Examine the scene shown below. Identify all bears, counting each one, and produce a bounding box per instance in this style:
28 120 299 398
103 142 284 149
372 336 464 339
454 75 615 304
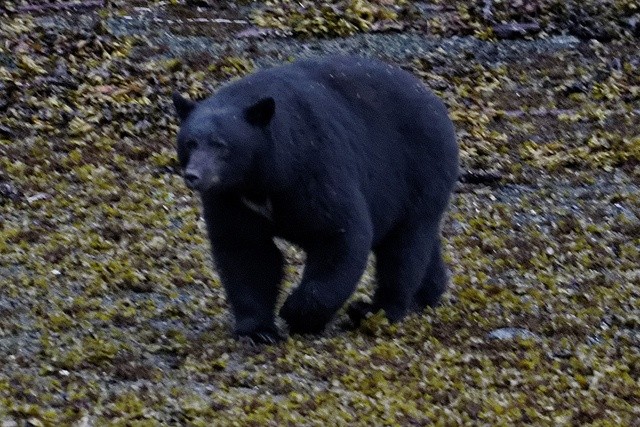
169 51 461 352
